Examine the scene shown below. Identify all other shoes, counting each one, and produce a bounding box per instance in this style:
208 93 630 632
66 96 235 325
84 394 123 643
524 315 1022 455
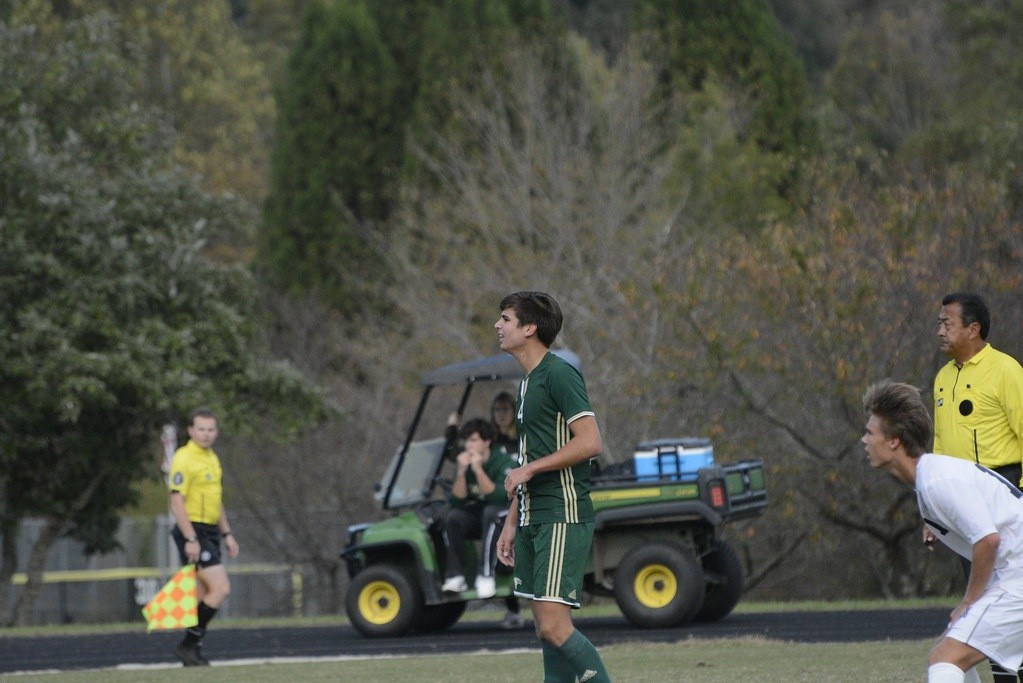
171 641 210 667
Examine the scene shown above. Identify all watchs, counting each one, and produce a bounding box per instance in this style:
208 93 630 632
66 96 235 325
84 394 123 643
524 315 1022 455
185 535 197 542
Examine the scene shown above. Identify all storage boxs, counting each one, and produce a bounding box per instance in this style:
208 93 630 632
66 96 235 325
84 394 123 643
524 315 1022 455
633 438 716 482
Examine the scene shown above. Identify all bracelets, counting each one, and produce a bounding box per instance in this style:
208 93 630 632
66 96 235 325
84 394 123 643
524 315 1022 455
222 531 232 539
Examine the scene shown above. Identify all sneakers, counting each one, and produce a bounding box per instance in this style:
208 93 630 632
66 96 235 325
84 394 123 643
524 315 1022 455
475 574 496 600
497 613 520 628
441 574 468 593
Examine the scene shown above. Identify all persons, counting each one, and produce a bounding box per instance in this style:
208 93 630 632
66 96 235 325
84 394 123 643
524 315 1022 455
495 291 612 683
858 378 1023 683
912 288 1023 683
435 391 528 630
167 406 238 669
